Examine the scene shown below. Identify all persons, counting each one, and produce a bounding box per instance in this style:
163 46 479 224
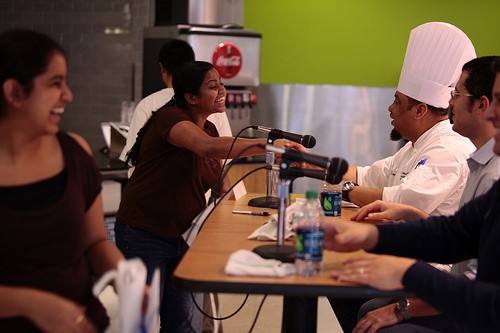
119 35 235 247
275 55 500 333
113 60 304 332
0 26 153 333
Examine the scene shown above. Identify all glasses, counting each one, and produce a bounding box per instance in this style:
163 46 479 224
449 90 473 99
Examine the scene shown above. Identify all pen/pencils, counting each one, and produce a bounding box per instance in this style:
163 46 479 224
232 210 271 216
414 159 425 170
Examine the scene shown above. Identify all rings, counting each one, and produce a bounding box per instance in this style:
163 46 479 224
74 313 84 324
359 267 364 275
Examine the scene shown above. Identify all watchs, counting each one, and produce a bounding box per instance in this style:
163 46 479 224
396 297 410 317
342 181 360 203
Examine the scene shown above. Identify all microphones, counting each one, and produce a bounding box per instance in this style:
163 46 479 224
265 143 348 173
266 163 343 185
252 124 316 148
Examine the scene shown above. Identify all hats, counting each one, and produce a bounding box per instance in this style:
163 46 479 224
396 22 478 110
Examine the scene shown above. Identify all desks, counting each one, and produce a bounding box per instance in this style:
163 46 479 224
175 162 417 333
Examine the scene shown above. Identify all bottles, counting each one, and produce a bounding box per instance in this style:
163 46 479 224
294 190 325 278
320 180 343 219
